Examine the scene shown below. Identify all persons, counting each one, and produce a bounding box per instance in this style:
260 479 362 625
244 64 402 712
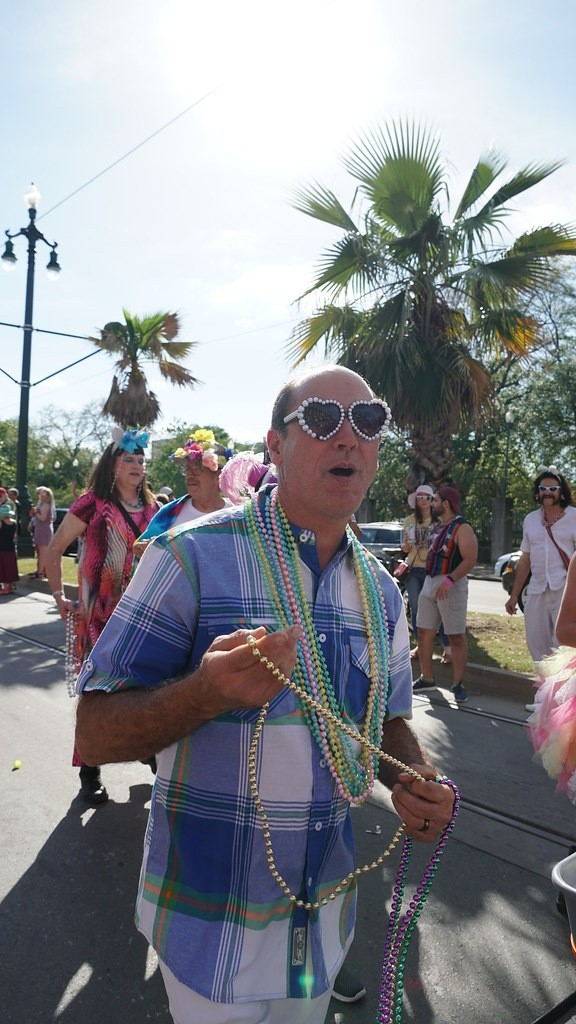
0 487 21 594
331 965 366 1002
28 486 56 579
524 552 576 807
505 471 575 714
73 362 456 1024
399 483 452 663
412 487 477 703
43 428 157 803
130 429 235 560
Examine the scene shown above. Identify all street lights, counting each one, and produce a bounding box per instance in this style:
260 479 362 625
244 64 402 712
0 179 62 559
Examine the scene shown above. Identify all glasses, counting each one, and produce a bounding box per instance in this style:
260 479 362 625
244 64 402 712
416 495 432 501
178 462 210 476
539 486 561 492
282 397 393 443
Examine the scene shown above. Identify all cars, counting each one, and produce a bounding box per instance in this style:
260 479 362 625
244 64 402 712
495 550 532 614
14 508 78 556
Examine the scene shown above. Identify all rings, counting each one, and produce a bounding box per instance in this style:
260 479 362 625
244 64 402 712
419 820 429 832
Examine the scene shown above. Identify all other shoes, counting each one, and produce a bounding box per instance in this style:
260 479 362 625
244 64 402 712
526 703 538 712
78 762 110 803
27 571 45 579
410 648 418 658
0 587 14 595
331 963 366 1003
441 652 452 663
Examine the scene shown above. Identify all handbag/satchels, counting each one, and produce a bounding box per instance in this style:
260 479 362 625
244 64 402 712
393 563 412 581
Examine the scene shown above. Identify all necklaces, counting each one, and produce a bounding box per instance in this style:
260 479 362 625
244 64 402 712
540 507 563 527
65 610 78 698
243 484 392 803
378 776 461 1023
430 517 452 553
244 634 428 912
414 518 432 562
121 494 143 509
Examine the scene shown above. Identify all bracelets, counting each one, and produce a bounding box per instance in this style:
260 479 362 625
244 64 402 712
52 590 64 597
443 577 453 587
447 575 455 582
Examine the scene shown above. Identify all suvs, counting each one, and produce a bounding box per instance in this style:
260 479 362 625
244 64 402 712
356 521 403 570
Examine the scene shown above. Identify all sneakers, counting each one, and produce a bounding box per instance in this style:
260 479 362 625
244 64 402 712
451 680 467 702
412 675 437 691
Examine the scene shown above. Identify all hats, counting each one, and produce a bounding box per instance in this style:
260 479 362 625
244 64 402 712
216 449 279 506
0 504 15 515
166 428 233 468
159 486 174 495
407 484 437 508
0 487 7 494
438 486 461 513
